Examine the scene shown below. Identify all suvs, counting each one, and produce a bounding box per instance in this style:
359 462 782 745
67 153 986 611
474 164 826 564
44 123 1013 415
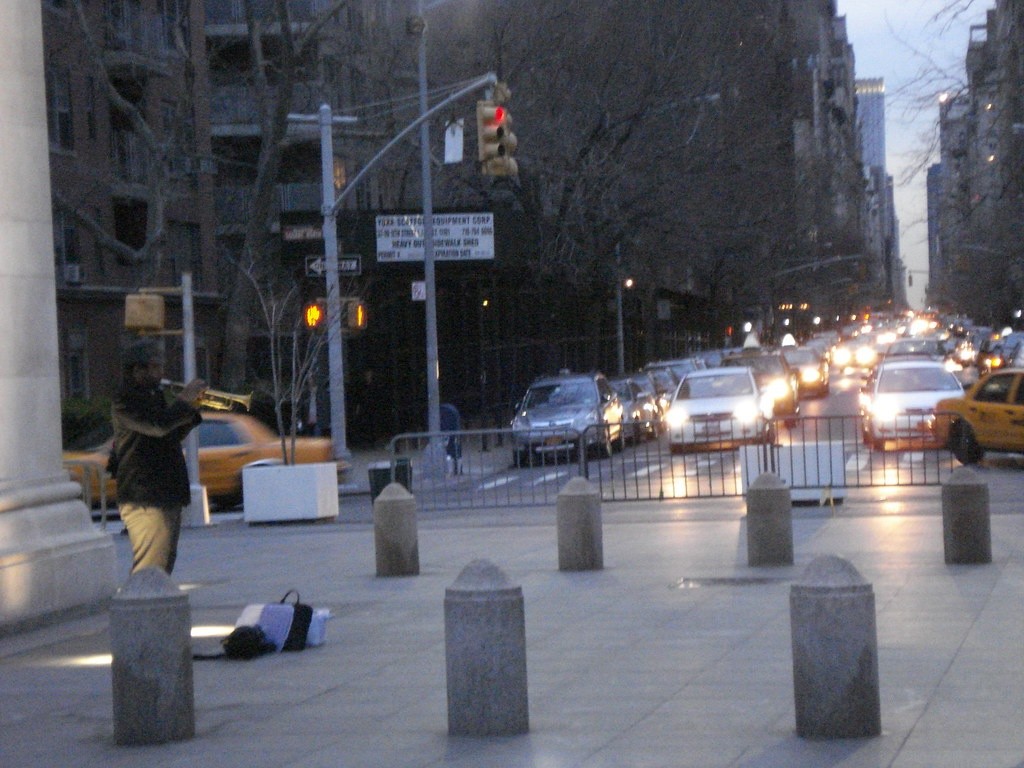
511 369 625 468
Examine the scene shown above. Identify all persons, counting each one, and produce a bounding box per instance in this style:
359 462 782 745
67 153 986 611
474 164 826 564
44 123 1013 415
441 398 467 475
105 338 214 580
480 364 504 453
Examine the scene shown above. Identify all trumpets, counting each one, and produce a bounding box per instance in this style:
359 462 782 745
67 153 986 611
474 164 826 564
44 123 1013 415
158 375 255 414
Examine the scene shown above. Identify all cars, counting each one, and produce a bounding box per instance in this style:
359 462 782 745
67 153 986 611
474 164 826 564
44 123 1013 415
64 408 333 510
928 366 1024 465
664 366 774 451
609 310 1024 441
856 361 965 449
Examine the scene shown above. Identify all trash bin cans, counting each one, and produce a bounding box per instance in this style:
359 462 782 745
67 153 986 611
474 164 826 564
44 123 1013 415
368 457 413 508
424 404 463 476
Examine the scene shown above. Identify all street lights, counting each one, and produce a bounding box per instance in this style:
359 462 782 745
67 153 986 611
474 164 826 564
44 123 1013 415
611 89 724 374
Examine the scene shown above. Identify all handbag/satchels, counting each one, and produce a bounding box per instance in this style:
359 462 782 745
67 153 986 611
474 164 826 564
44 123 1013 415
258 590 312 649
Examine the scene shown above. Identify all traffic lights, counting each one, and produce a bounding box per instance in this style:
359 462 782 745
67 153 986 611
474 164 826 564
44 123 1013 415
304 303 323 331
474 99 506 165
347 302 367 329
481 112 520 178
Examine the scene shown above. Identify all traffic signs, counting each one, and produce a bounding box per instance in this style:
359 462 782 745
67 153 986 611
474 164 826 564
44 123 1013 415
303 252 363 277
